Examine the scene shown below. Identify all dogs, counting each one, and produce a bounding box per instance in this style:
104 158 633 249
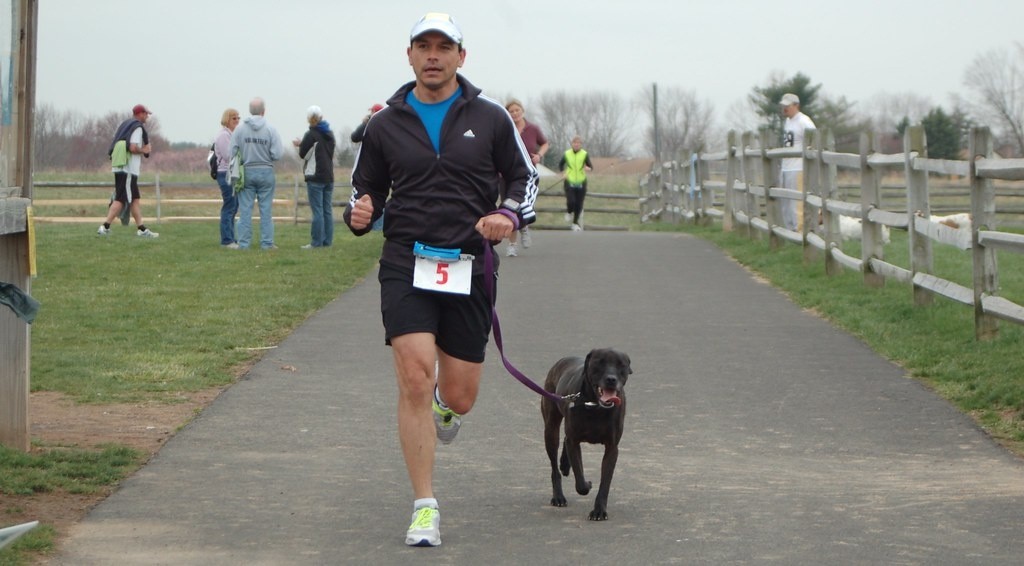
541 348 633 521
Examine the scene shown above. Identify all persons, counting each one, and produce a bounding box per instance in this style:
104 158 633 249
777 93 817 234
558 134 593 231
230 97 282 249
214 108 238 248
342 13 538 547
499 98 549 257
292 106 336 248
97 104 159 238
350 104 383 231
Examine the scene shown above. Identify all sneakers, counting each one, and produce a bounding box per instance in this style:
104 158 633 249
520 230 531 248
505 246 517 257
97 225 113 236
138 228 160 238
405 507 442 546
431 380 462 445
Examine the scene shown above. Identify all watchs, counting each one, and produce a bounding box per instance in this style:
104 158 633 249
499 198 521 214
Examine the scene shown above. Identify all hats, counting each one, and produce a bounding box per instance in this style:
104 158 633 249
134 104 153 115
779 93 799 105
409 13 463 44
369 104 383 111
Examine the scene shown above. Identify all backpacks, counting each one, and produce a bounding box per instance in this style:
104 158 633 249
208 143 222 180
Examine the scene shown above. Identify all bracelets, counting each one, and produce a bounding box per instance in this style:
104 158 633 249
534 152 541 157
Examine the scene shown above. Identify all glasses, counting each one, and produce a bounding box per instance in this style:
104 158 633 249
232 117 240 120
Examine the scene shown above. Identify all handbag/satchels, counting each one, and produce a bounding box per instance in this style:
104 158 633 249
111 141 130 169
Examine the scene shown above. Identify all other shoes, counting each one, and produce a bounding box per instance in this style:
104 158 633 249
564 213 572 223
572 223 580 231
222 243 239 249
300 245 312 249
